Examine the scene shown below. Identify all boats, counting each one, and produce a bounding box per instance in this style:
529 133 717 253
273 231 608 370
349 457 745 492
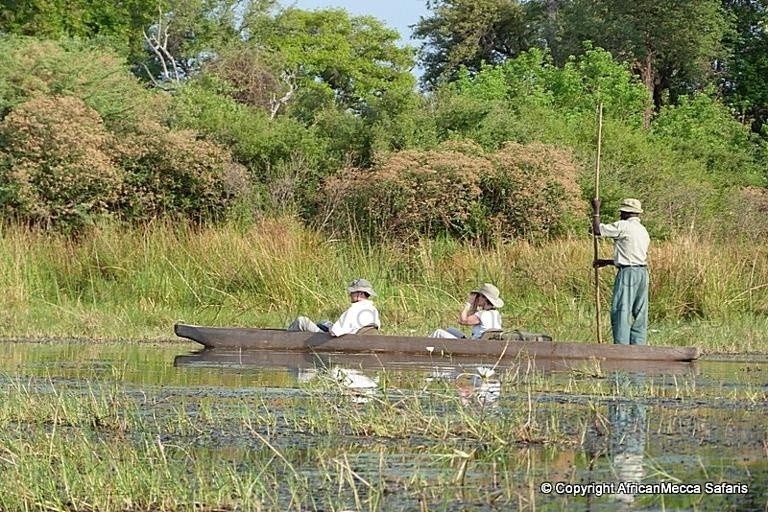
173 320 704 367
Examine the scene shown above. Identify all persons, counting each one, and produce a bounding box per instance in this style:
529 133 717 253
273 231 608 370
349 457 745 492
288 279 380 338
591 198 650 346
429 283 504 339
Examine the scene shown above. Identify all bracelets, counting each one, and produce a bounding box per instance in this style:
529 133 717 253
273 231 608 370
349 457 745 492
593 213 599 217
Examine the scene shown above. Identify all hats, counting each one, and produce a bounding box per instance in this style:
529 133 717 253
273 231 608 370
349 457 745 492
618 199 644 214
470 283 504 308
347 279 377 296
